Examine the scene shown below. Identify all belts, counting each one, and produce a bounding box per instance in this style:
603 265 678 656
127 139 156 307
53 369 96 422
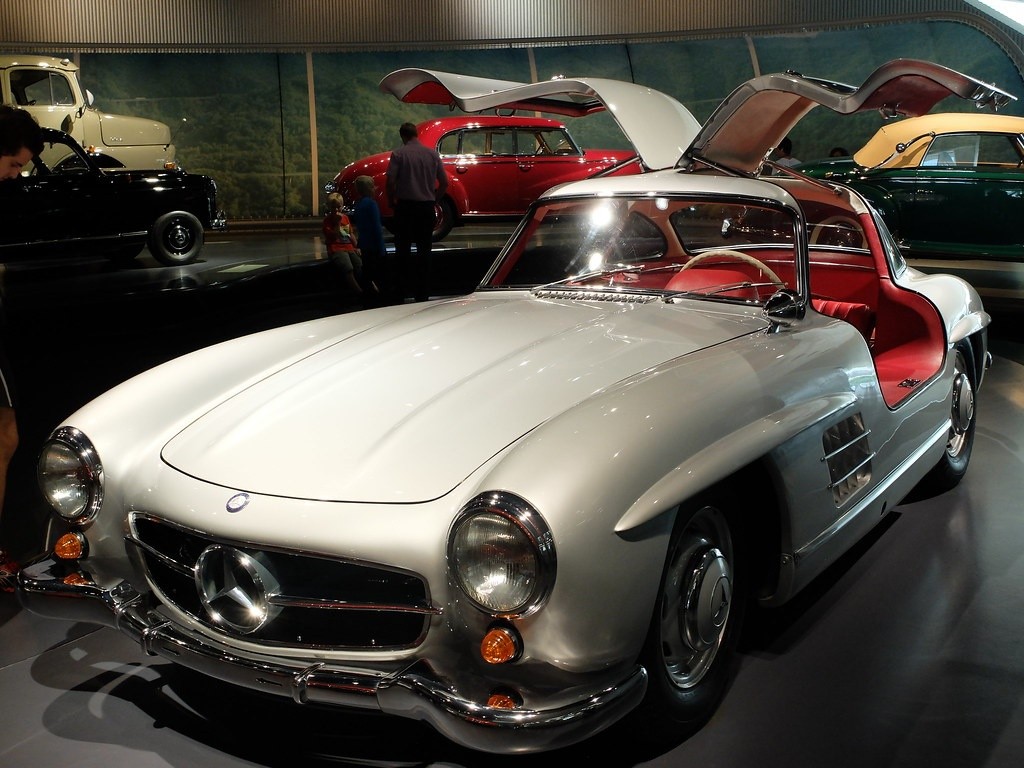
398 199 436 205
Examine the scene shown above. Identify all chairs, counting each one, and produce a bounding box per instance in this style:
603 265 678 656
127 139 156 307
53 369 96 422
811 299 876 345
937 152 955 166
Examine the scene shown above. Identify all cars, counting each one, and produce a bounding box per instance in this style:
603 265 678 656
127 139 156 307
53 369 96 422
0 53 177 174
323 112 1024 306
0 126 228 278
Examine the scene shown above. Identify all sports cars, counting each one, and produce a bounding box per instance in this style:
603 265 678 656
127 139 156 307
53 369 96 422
25 57 1020 763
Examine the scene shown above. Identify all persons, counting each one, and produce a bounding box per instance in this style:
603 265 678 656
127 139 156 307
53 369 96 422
829 147 850 157
345 175 386 296
772 138 802 175
0 104 68 592
385 123 448 303
323 192 363 294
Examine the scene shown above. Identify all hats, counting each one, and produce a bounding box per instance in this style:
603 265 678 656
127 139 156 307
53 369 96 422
355 175 373 193
328 192 343 206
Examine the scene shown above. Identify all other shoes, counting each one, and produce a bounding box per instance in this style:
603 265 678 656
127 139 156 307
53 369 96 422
0 549 18 592
